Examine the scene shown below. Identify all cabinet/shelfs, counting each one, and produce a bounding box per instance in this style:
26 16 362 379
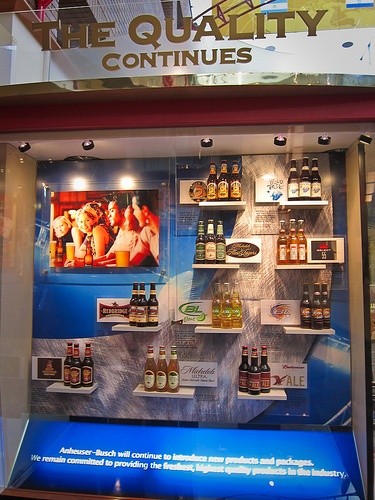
46 200 336 401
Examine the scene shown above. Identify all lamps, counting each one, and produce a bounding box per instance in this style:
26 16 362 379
201 137 213 147
274 135 287 145
18 142 30 153
358 132 373 145
82 140 94 150
318 132 334 146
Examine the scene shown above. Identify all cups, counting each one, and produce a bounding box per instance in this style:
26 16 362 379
66 242 75 261
115 245 130 267
50 241 57 259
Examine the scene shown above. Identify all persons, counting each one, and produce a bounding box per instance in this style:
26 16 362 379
52 192 159 266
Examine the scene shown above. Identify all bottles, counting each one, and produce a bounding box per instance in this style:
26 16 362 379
57 239 64 263
144 345 180 392
277 218 307 265
64 342 94 387
207 160 242 201
300 282 330 330
85 236 93 266
287 157 322 201
128 282 159 326
195 218 226 264
238 346 270 395
213 282 243 329
